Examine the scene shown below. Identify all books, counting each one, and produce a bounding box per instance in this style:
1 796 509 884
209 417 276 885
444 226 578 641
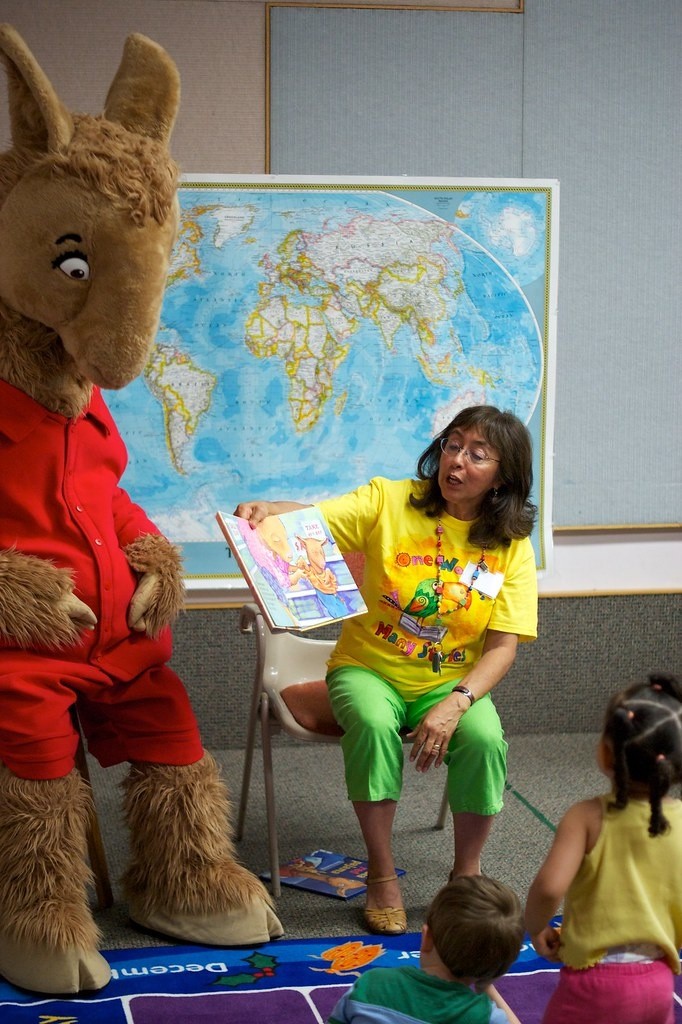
215 504 368 634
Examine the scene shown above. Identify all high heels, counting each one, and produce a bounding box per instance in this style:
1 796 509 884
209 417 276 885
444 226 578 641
362 874 408 935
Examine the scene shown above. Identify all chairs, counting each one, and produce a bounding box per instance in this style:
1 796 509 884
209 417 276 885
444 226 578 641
234 602 448 898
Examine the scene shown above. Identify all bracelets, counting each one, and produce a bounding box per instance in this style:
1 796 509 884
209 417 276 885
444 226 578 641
452 686 476 707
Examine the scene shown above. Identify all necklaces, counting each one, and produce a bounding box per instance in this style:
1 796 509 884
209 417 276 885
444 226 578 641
432 507 487 644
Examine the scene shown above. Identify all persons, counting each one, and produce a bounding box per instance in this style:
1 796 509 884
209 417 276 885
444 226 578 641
523 668 682 1024
318 875 526 1024
236 402 540 938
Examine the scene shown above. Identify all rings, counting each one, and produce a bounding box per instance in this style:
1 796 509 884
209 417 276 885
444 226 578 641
433 745 441 751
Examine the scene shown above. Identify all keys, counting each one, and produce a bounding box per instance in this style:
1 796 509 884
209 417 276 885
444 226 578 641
437 652 443 677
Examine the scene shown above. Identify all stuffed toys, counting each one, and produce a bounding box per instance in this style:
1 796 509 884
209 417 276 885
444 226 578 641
0 23 288 1002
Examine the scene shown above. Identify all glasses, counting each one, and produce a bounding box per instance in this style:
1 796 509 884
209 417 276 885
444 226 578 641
440 437 501 465
